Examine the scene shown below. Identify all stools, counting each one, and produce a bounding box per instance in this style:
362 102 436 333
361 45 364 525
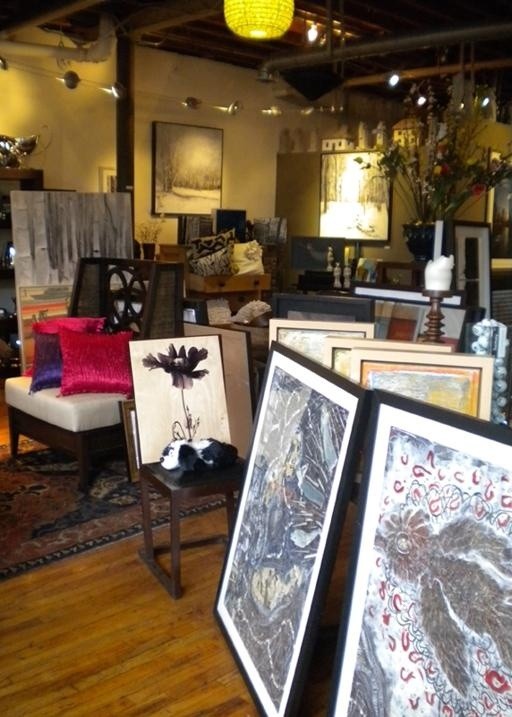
138 453 248 598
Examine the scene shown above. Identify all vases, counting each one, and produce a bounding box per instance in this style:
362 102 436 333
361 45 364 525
403 220 436 260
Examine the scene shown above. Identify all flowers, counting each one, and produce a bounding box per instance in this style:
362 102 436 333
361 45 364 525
355 117 512 222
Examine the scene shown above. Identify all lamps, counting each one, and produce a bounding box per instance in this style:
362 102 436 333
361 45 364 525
390 75 400 86
307 24 319 41
416 97 426 106
224 0 295 38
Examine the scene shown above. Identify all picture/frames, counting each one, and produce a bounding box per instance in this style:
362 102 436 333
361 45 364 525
322 390 512 716
10 191 134 375
324 336 453 380
319 149 394 241
130 333 234 468
152 121 223 215
213 342 369 717
351 348 494 422
269 318 374 368
375 299 474 354
272 293 375 322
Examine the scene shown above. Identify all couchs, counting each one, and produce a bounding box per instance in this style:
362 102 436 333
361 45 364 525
5 256 184 494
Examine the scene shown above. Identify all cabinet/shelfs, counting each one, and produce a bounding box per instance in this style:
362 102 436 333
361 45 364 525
0 169 44 375
